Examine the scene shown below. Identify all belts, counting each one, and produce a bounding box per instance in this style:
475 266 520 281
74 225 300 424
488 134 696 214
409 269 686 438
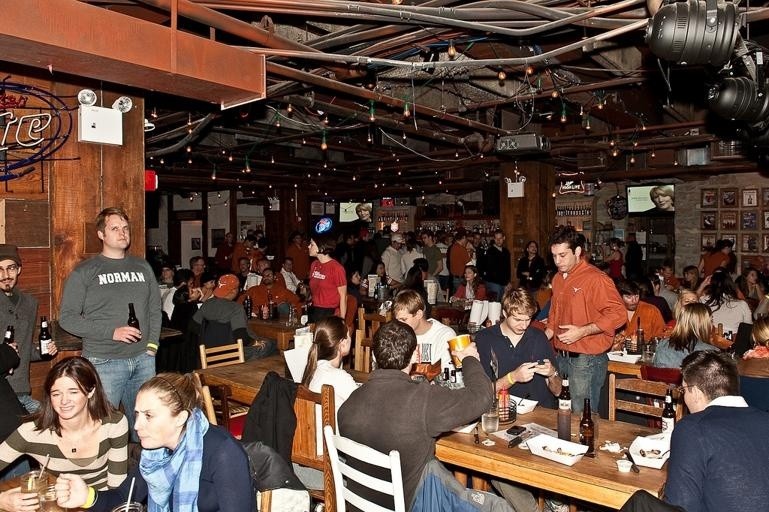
554 347 584 360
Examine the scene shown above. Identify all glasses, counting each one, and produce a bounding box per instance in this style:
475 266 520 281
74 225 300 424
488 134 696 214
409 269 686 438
675 383 696 394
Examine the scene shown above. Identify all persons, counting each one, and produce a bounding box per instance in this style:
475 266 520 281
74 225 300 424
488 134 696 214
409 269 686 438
472 285 572 512
335 320 516 512
53 371 258 511
639 183 674 212
1 355 129 491
58 205 163 444
1 242 59 419
148 215 768 419
0 485 42 512
0 337 33 482
290 314 365 492
347 203 373 222
618 348 768 511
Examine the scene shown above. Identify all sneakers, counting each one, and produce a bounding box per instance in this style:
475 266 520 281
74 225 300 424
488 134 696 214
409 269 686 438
543 498 570 512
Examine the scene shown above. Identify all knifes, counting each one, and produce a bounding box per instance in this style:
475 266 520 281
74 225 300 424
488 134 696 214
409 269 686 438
474 426 479 444
508 430 532 448
624 451 640 473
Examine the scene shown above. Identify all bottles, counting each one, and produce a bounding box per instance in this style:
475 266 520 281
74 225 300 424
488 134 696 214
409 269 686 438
4 325 18 376
584 238 591 251
579 398 595 452
128 303 142 343
557 402 571 442
372 281 392 301
556 203 591 217
717 323 733 352
38 315 53 360
661 389 675 434
288 304 309 326
443 368 462 385
592 254 596 261
378 211 408 221
420 221 499 234
498 385 511 421
244 294 252 319
558 374 571 412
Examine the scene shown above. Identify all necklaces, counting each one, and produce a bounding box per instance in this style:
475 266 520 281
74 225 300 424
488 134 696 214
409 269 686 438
51 418 103 452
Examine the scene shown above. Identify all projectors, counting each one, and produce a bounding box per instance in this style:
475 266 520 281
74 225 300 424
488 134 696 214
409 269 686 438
497 134 552 155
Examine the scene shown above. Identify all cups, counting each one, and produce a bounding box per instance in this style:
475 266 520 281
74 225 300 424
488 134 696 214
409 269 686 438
466 299 502 333
616 459 633 473
19 471 51 512
625 328 661 365
110 502 147 512
447 335 470 368
38 484 67 512
481 412 499 435
259 302 278 320
580 411 599 448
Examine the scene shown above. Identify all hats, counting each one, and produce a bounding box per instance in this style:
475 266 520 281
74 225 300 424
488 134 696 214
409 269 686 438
0 242 23 268
161 263 177 276
390 232 406 244
212 273 241 299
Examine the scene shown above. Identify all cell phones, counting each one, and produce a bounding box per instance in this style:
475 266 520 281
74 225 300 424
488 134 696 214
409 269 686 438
507 424 527 435
531 360 544 370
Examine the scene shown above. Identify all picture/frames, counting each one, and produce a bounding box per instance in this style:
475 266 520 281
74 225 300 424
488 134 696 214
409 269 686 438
695 184 769 276
